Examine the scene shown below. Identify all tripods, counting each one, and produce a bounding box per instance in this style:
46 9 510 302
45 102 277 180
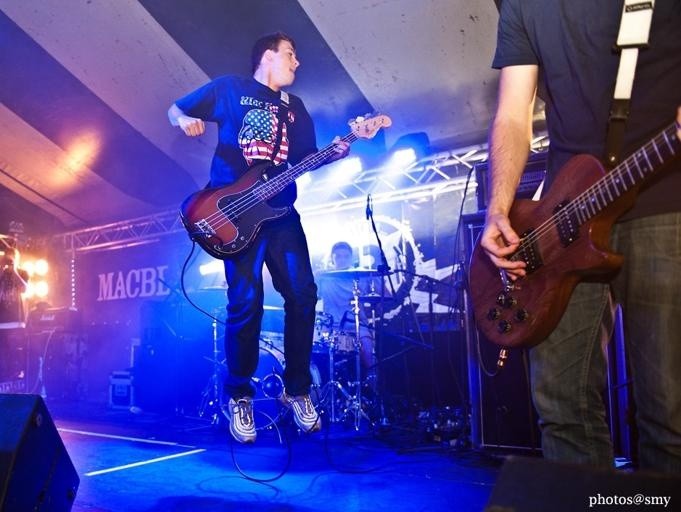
199 296 375 431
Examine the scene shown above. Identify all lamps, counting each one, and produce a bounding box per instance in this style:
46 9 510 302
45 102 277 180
330 144 372 176
389 132 432 166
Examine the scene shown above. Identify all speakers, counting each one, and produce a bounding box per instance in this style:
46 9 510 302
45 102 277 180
374 310 471 406
0 393 81 511
476 330 537 449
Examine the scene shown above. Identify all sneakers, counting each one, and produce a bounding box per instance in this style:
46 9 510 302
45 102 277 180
229 396 257 444
280 387 321 433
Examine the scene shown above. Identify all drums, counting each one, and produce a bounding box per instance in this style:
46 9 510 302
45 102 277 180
259 305 285 339
322 330 356 356
211 338 323 433
310 308 333 355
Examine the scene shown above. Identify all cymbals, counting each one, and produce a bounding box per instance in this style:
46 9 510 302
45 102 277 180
351 293 393 304
321 269 379 277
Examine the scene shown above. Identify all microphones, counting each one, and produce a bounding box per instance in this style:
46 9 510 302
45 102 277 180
365 197 371 220
496 346 511 371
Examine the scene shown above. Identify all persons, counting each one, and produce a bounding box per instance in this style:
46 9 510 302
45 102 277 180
316 242 390 380
167 34 350 443
0 247 29 378
481 0 681 475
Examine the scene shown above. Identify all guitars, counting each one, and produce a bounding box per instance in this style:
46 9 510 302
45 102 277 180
467 116 681 349
180 112 393 260
